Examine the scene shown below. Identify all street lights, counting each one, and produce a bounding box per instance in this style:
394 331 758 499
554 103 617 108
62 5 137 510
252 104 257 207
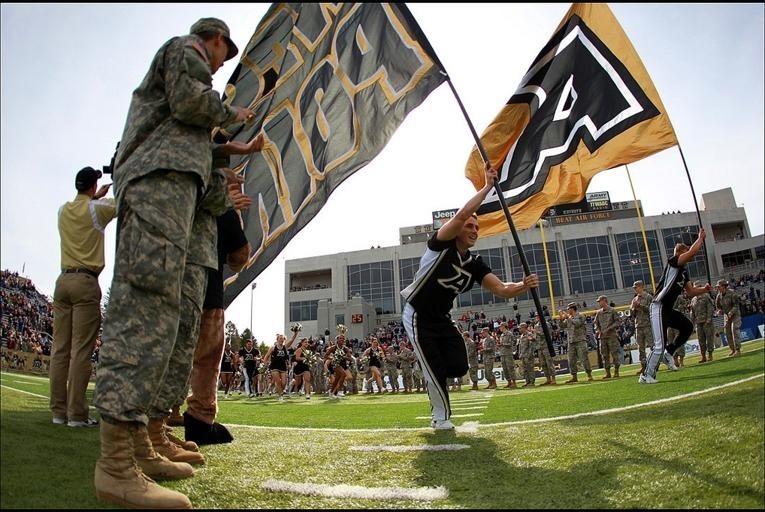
248 281 258 349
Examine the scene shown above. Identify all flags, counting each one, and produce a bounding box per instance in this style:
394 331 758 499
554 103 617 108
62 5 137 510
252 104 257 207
211 2 449 310
464 1 678 240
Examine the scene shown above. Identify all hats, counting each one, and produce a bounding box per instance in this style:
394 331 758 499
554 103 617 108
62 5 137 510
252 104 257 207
479 278 731 334
188 17 239 61
74 166 102 191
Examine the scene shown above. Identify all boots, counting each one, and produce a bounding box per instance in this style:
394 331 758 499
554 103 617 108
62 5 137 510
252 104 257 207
147 419 206 466
94 416 194 509
159 420 200 453
469 347 743 391
128 420 193 481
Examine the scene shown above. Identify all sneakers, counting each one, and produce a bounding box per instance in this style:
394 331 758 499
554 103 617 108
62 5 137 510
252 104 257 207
662 352 678 371
638 372 659 385
52 414 100 427
430 417 456 431
